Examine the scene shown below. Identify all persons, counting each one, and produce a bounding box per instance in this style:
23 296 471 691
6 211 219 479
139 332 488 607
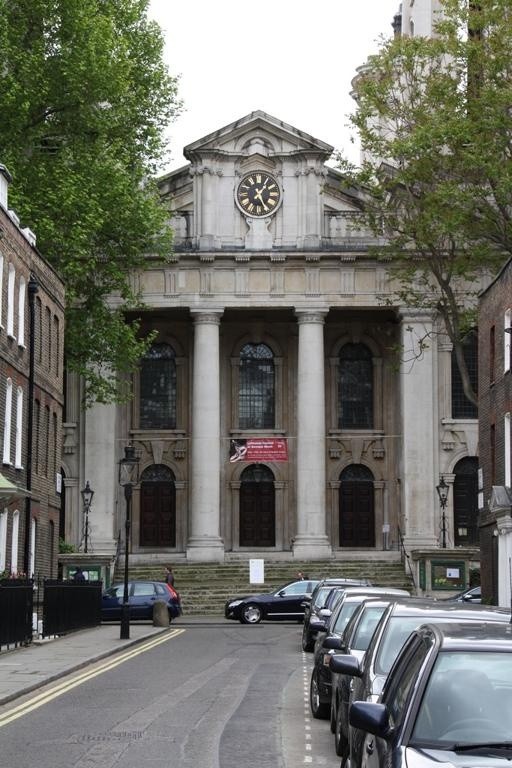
295 571 305 580
75 568 85 579
164 566 175 587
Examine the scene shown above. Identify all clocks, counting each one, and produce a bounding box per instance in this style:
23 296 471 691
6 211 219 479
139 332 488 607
232 168 284 220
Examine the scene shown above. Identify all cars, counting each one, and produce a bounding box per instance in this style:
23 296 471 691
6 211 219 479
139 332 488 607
101 580 183 624
320 600 395 758
222 576 373 625
445 586 482 603
346 623 512 767
301 582 412 719
330 599 511 768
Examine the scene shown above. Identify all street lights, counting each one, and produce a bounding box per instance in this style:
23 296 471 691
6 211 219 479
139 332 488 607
80 480 94 553
117 441 141 639
435 475 450 548
